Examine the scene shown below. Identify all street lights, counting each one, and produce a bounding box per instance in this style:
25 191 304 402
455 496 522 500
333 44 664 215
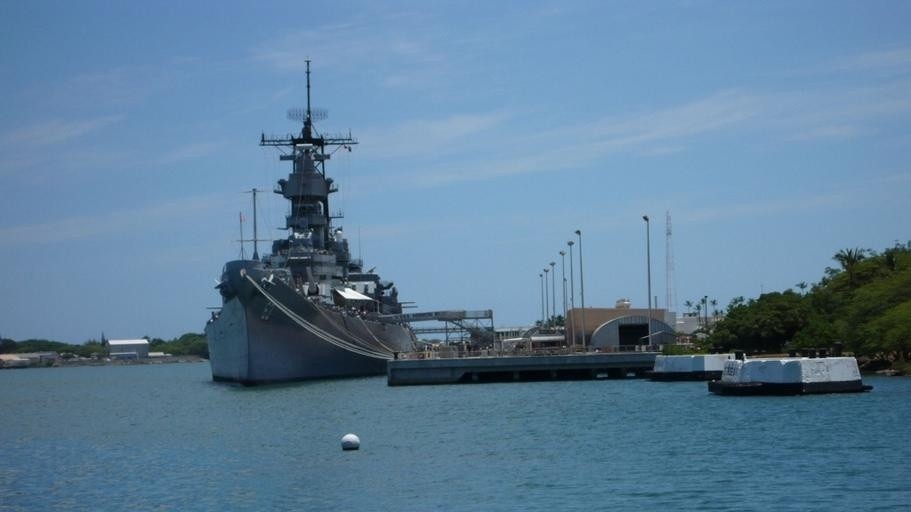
642 215 653 350
538 229 587 351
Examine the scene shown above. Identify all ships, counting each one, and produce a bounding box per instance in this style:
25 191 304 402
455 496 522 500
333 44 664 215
203 57 425 385
